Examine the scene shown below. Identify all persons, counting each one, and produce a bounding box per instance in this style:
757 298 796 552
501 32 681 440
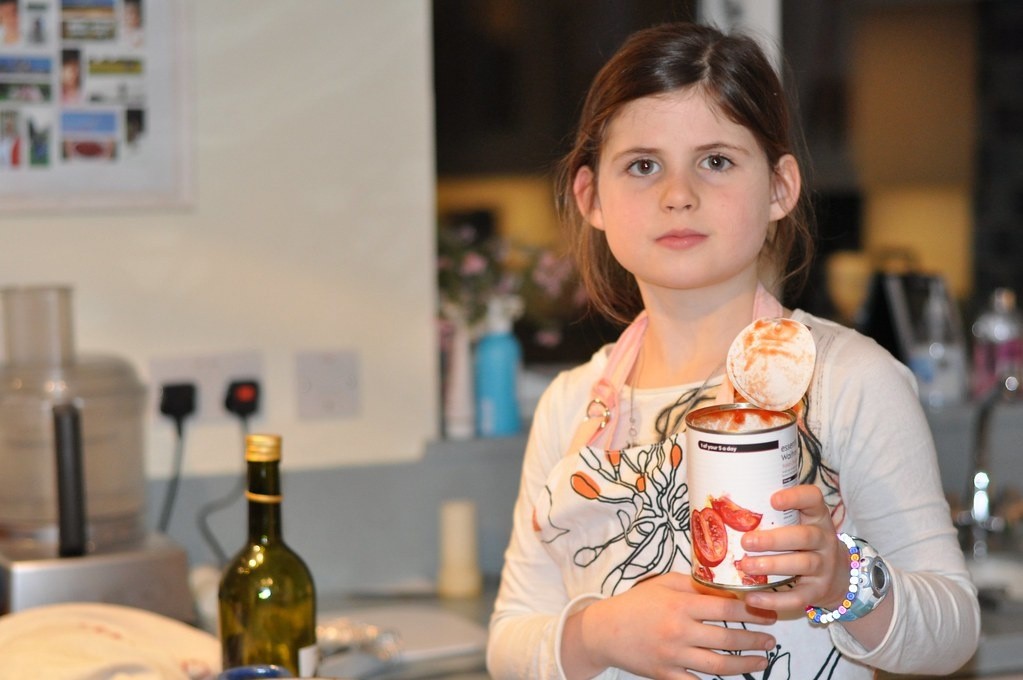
485 24 981 680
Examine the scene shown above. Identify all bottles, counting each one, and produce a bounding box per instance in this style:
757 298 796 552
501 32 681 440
909 277 965 403
218 435 316 680
474 294 520 434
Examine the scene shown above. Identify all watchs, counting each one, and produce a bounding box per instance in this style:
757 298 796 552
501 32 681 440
821 535 891 621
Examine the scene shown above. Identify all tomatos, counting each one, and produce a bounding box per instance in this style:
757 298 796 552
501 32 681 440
690 495 764 568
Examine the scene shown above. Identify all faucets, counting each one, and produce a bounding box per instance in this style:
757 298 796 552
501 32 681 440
962 370 1020 560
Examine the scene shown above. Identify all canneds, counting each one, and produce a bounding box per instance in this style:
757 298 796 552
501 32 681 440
684 400 801 595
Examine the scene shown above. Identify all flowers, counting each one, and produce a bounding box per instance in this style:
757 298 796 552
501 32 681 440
437 228 592 352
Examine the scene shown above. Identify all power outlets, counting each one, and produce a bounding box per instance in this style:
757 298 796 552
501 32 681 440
154 349 263 430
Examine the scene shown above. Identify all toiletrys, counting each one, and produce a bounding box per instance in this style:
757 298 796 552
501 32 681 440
473 292 526 437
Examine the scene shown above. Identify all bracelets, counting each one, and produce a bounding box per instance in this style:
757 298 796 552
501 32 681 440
804 533 860 624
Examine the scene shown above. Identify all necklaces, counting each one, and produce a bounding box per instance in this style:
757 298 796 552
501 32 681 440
630 341 726 447
